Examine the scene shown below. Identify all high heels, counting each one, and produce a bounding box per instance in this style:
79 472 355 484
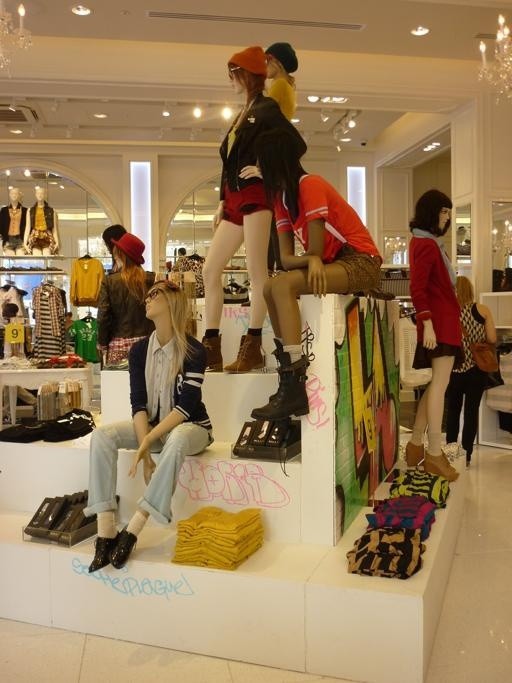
406 442 426 466
424 451 459 481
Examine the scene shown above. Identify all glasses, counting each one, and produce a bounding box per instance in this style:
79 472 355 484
145 288 159 299
228 66 240 79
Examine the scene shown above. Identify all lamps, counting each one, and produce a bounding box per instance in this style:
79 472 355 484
491 215 512 270
319 111 357 134
0 0 34 80
477 13 512 108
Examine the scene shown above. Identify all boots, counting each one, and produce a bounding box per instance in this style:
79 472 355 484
251 339 310 419
201 333 222 372
225 334 265 373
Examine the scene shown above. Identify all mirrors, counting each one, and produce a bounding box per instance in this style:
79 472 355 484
381 230 410 265
452 192 472 283
489 199 512 292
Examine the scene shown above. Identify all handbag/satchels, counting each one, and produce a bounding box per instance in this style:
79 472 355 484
470 342 499 373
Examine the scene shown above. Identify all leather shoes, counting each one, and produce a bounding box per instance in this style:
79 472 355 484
109 524 137 569
88 531 119 573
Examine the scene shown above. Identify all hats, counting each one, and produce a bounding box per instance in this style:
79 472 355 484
268 42 297 73
111 233 145 264
228 46 267 76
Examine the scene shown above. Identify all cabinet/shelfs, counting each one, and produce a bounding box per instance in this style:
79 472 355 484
0 253 66 276
0 290 468 683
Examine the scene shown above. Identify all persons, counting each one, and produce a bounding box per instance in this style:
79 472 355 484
102 224 127 267
166 261 172 280
2 303 41 424
264 42 298 277
23 186 59 255
199 46 308 374
31 310 35 351
445 276 497 469
250 127 383 422
97 232 156 370
0 188 27 257
63 311 77 331
83 280 215 573
178 248 186 256
404 189 460 480
